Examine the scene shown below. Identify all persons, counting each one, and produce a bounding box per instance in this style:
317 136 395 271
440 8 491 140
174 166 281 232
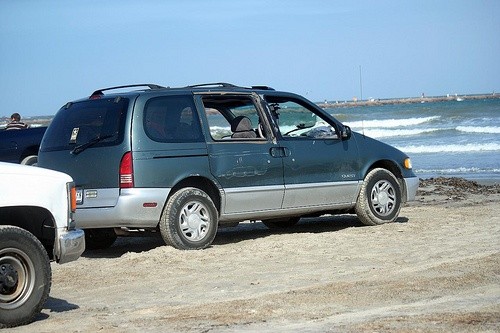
5 112 30 131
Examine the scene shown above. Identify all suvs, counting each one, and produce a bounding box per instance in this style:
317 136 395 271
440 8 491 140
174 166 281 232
36 82 420 249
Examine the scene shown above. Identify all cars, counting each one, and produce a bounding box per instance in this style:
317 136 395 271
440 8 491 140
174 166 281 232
0 161 86 329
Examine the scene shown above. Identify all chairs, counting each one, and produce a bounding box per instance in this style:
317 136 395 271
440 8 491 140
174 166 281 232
231 116 257 137
